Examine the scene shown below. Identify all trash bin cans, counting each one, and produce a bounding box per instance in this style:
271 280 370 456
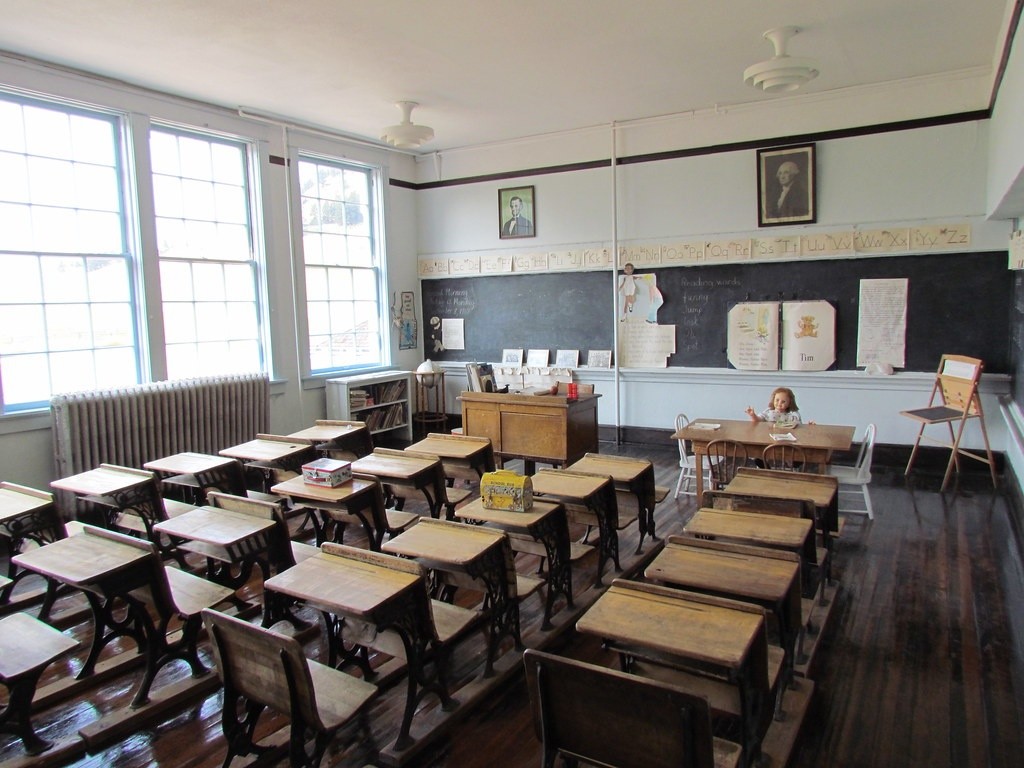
451 428 463 436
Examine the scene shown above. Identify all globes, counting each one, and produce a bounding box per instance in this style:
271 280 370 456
416 359 442 389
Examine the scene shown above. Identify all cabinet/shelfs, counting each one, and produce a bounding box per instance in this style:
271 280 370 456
455 387 603 470
325 370 413 441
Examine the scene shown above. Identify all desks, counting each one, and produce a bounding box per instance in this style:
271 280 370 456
153 505 278 630
722 471 838 586
455 496 571 640
404 437 493 489
575 583 768 768
144 451 241 496
264 551 432 768
0 487 54 616
218 439 313 470
286 425 373 459
271 471 382 552
11 530 171 748
644 540 800 721
684 506 813 554
564 456 653 567
50 466 161 542
381 519 507 705
351 452 441 518
670 417 855 510
530 466 616 599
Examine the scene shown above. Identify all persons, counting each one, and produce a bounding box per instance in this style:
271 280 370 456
503 197 532 235
770 161 807 217
745 388 816 469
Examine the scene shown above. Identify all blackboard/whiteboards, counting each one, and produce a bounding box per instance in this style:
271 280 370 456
996 268 1024 454
419 249 1012 380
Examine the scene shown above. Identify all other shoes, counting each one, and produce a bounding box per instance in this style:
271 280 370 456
754 458 771 469
792 461 803 468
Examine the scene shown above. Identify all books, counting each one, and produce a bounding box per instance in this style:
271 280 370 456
688 423 721 430
773 422 798 428
770 433 797 441
466 362 498 392
520 386 551 396
350 381 406 431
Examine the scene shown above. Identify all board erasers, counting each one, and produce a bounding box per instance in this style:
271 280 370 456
864 363 894 376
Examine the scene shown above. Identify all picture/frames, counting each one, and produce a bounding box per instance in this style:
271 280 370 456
498 184 537 240
756 141 816 227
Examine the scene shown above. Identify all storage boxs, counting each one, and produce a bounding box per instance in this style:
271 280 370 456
301 458 353 489
479 469 534 512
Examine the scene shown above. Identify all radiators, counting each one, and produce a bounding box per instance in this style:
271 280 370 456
51 371 272 520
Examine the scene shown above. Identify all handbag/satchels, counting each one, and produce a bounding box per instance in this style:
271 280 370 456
479 469 534 513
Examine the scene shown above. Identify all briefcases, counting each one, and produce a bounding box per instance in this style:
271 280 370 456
301 457 353 489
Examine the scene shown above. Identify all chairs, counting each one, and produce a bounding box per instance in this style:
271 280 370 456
0 417 848 768
525 380 594 476
900 351 1000 490
670 413 878 519
